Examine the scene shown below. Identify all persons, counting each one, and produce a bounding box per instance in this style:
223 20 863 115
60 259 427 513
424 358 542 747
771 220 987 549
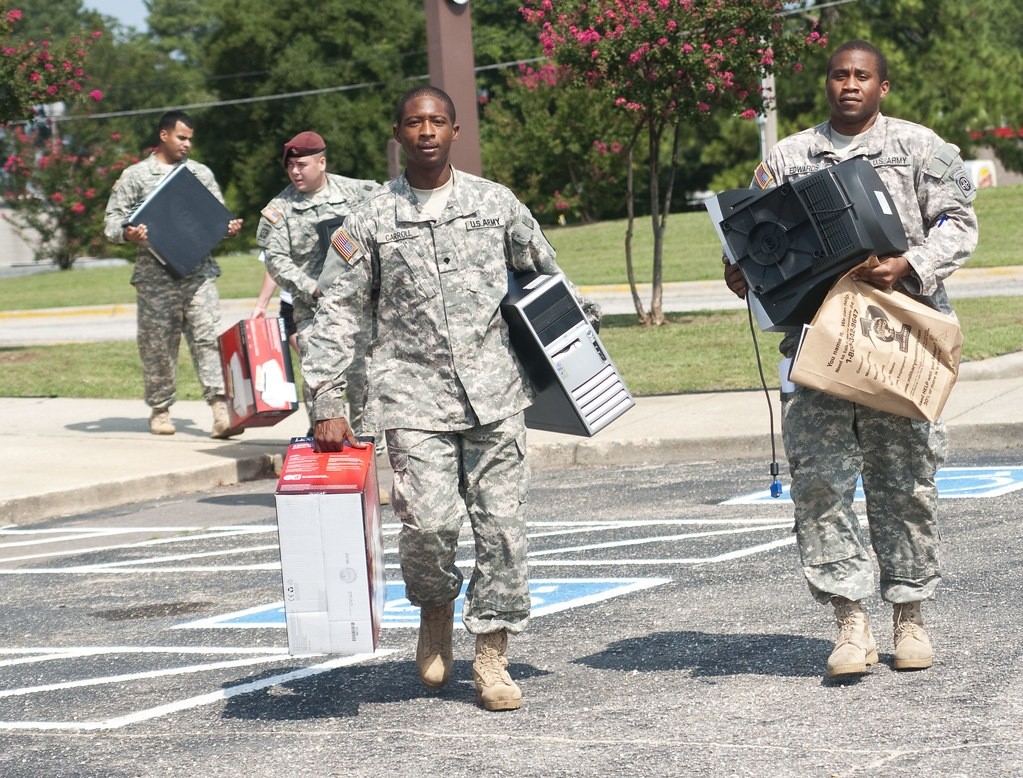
299 85 602 711
718 38 979 683
255 131 392 505
251 250 313 437
102 110 244 439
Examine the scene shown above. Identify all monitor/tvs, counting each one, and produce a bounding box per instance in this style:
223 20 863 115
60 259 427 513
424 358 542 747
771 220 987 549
704 158 909 334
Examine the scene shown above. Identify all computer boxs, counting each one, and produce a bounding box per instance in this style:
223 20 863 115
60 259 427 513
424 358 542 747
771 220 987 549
498 269 634 438
120 164 236 284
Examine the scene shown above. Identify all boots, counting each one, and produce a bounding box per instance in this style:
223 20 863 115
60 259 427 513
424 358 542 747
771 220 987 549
148 406 176 434
211 397 245 439
827 594 879 677
414 599 457 687
888 599 936 670
473 629 524 711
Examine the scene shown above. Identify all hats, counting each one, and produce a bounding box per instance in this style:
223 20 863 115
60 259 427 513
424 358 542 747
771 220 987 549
281 130 326 169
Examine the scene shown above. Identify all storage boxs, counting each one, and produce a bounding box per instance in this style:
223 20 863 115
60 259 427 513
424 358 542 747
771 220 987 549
216 316 300 430
274 435 388 655
